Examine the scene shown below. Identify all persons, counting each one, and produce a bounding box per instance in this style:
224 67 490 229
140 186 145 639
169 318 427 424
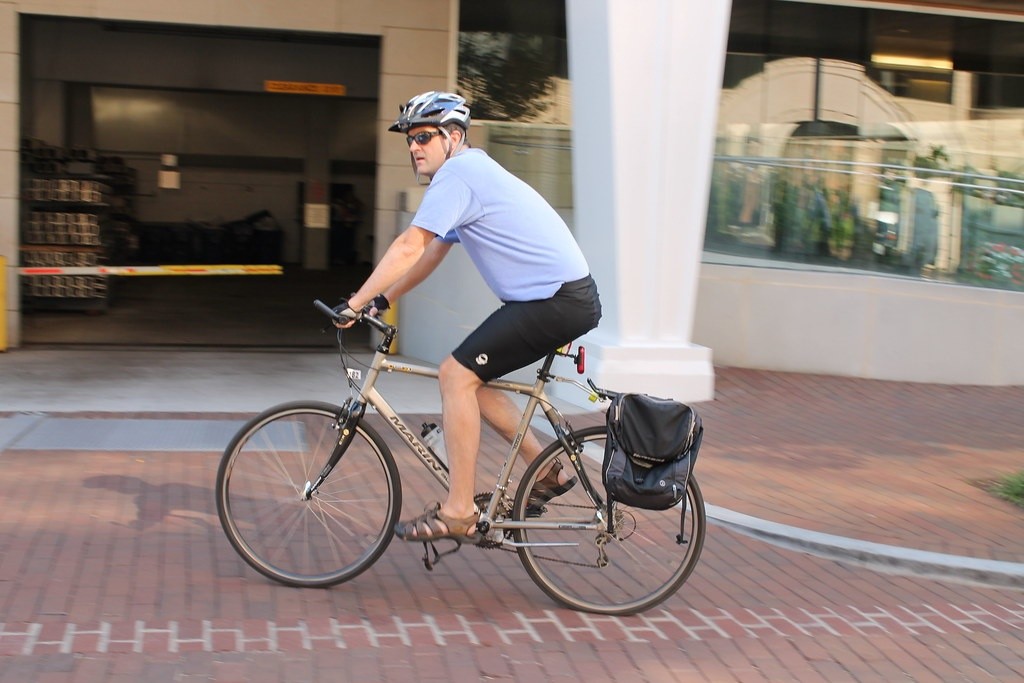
331 90 603 542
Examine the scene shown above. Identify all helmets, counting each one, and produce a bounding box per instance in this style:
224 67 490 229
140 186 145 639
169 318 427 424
388 90 472 132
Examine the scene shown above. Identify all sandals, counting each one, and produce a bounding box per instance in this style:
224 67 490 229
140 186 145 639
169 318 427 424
394 500 483 544
524 461 579 518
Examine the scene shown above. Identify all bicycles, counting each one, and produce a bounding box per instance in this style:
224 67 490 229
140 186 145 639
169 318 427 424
215 296 708 617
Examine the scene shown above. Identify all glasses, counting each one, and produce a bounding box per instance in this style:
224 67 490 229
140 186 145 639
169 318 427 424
406 130 444 145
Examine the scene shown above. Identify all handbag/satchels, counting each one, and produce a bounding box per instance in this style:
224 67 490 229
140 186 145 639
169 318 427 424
601 392 704 512
